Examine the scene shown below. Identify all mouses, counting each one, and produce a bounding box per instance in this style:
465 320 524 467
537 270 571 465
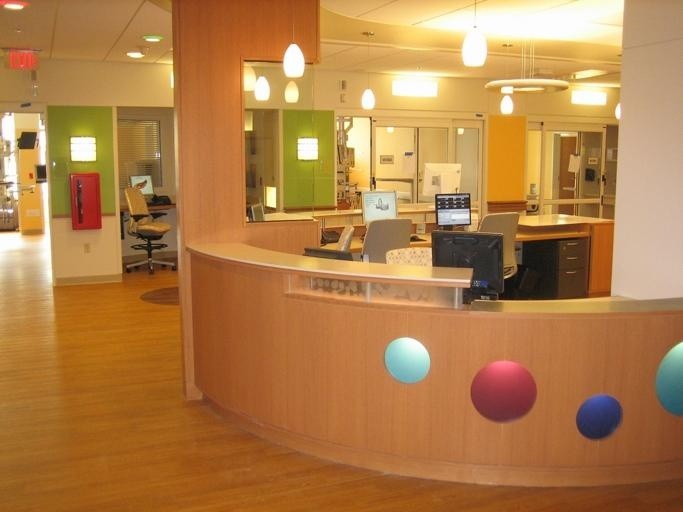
410 235 420 241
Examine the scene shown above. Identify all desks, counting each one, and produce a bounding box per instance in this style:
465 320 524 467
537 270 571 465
306 209 615 298
120 200 176 222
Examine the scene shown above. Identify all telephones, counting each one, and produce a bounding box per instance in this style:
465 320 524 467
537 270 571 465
321 229 340 244
151 195 171 206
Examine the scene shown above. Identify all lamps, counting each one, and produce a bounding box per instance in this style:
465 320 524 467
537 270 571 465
254 76 270 100
242 62 256 91
499 94 513 114
360 30 376 111
460 0 487 68
282 0 304 78
284 80 299 103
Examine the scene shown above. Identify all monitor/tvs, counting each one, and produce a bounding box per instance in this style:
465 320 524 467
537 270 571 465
248 203 265 221
361 190 399 226
435 193 471 231
19 132 37 149
422 163 462 197
36 165 46 183
302 247 362 296
431 231 504 304
129 174 155 199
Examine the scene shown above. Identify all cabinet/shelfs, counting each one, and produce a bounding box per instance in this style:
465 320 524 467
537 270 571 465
523 239 589 299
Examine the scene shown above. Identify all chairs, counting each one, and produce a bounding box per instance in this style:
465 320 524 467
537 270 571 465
122 187 176 275
383 246 431 266
359 217 412 262
477 212 519 281
336 224 355 252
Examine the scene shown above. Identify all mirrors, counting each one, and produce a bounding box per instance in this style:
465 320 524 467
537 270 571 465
242 60 316 223
